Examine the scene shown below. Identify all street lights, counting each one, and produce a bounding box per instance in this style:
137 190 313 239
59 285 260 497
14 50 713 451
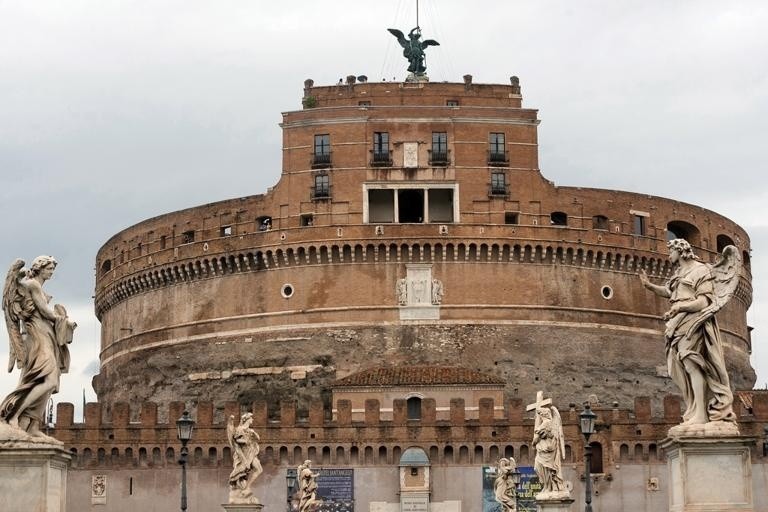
176 407 195 510
579 402 597 511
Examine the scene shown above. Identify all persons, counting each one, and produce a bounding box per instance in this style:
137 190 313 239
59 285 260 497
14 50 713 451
307 218 311 226
408 26 425 73
639 238 714 425
495 458 511 509
260 223 271 231
531 408 553 492
234 413 263 490
301 460 321 512
431 275 439 302
8 255 77 445
400 276 407 303
